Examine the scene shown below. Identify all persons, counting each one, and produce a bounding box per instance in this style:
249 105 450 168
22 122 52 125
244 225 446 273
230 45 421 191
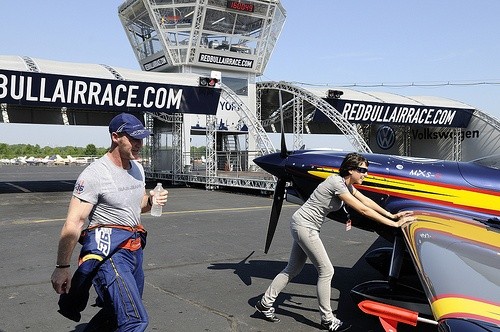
255 152 416 332
51 114 168 332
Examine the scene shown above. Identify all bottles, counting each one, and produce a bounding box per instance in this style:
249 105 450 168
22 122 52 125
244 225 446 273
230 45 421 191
150 183 164 217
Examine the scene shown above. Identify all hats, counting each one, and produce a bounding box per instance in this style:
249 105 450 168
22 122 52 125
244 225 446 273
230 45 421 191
109 113 154 140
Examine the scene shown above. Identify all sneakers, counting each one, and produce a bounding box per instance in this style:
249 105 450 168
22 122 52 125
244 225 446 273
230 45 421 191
320 316 352 332
255 300 281 323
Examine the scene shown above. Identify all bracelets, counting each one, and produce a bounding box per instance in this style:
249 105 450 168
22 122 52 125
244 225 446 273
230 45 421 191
56 264 70 268
148 196 152 206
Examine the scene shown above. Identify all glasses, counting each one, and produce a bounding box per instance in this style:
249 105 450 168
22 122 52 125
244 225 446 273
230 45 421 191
355 166 368 174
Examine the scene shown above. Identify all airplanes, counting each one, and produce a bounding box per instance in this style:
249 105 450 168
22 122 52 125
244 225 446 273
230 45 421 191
251 126 500 332
